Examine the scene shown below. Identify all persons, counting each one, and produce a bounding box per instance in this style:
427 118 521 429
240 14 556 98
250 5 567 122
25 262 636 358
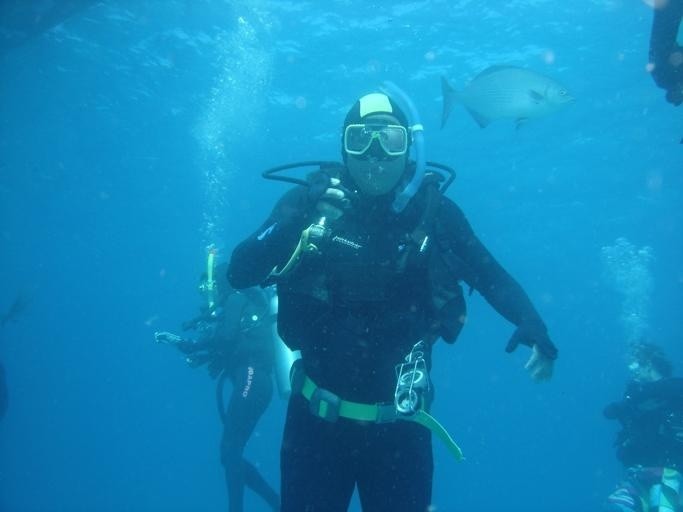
228 90 558 511
155 264 281 511
608 348 683 511
648 1 683 106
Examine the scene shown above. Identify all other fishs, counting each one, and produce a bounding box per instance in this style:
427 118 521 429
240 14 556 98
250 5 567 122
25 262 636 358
440 65 577 130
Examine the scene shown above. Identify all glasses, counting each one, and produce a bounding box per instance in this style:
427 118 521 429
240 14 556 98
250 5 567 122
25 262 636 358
343 125 408 157
